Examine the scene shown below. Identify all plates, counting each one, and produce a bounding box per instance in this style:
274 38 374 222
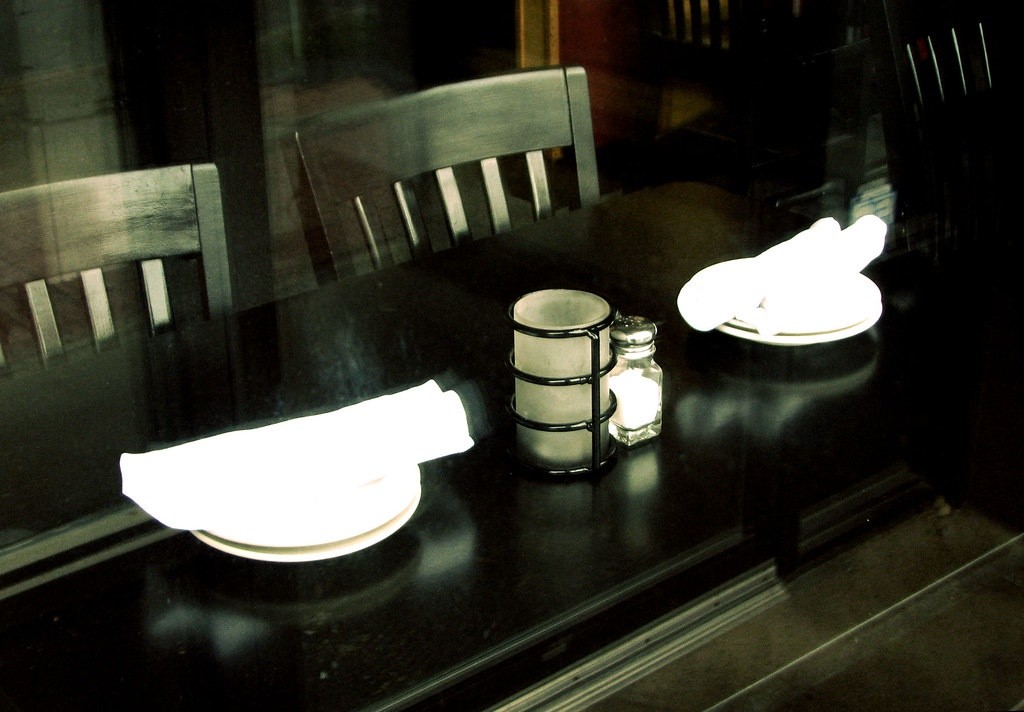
189 465 421 562
717 271 883 346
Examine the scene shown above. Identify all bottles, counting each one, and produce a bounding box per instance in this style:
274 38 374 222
608 314 664 446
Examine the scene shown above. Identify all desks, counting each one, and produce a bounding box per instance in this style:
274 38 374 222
0 182 1024 712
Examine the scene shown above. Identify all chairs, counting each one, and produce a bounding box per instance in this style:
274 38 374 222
293 65 601 287
0 161 233 369
862 0 995 266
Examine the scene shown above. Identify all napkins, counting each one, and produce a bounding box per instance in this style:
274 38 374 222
677 216 887 335
120 380 475 530
